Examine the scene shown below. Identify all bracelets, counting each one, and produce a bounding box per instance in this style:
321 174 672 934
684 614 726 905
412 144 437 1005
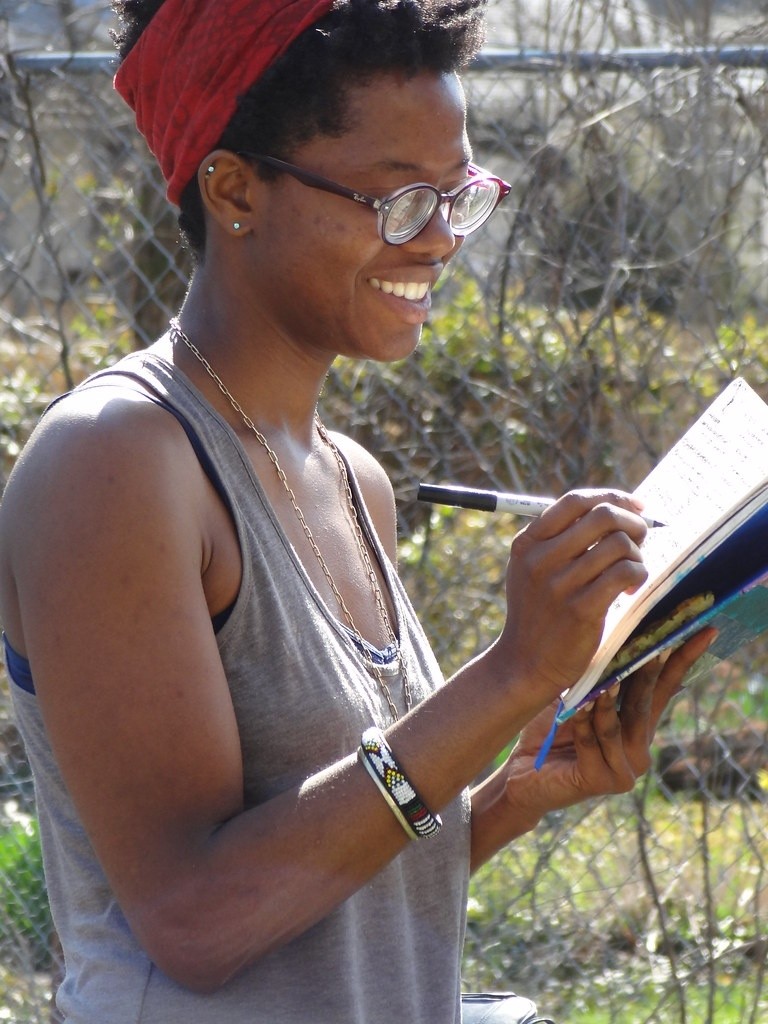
356 726 444 840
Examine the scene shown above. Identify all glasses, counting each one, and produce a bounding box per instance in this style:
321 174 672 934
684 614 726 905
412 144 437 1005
242 152 512 246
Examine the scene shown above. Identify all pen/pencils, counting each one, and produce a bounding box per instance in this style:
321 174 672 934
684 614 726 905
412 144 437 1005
415 484 671 529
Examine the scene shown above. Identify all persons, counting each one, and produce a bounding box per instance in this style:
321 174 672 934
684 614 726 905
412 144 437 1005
0 0 718 1024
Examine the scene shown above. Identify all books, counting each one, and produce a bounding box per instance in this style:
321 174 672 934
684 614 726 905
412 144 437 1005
541 377 768 726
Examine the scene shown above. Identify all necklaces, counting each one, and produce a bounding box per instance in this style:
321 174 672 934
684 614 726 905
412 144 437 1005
170 314 414 723
